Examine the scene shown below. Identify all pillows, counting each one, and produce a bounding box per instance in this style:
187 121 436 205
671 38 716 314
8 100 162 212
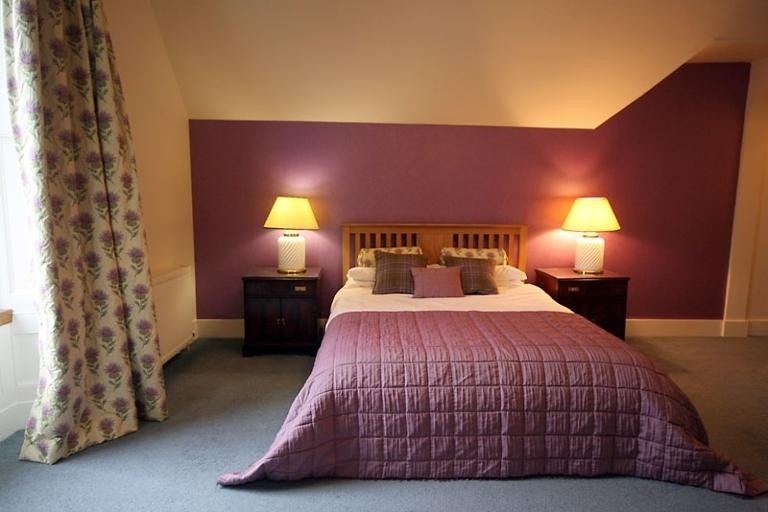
345 247 527 299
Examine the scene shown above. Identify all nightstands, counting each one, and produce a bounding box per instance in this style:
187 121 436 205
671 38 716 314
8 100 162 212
534 268 631 343
241 266 322 357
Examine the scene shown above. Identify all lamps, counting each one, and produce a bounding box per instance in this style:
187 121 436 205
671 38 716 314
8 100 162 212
263 196 321 275
561 196 621 274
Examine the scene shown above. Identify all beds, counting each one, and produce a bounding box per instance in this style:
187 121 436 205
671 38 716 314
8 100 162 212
217 222 768 498
